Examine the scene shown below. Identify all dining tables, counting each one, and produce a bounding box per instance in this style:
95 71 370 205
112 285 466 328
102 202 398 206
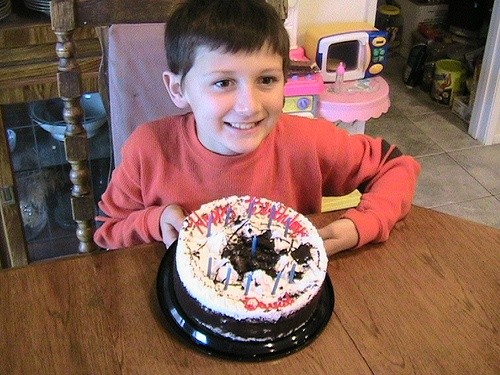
0 205 500 375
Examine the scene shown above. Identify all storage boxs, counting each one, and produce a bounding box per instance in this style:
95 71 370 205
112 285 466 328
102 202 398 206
451 95 472 124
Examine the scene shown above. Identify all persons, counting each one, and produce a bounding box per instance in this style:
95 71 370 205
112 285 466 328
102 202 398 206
93 0 421 257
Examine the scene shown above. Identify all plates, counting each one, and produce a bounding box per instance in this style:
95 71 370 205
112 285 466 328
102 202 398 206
156 234 334 362
0 0 50 20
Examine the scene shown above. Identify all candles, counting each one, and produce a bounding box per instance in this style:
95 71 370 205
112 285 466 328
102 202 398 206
207 197 297 295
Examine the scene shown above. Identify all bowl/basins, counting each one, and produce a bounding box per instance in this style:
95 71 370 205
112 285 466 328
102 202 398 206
29 93 107 141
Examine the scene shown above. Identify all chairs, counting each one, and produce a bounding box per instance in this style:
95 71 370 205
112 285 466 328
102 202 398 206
97 22 193 173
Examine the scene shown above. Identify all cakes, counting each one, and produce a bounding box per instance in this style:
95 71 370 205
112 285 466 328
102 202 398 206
171 194 328 342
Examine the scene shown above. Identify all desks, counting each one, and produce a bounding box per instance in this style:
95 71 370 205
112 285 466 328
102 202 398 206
320 75 392 137
